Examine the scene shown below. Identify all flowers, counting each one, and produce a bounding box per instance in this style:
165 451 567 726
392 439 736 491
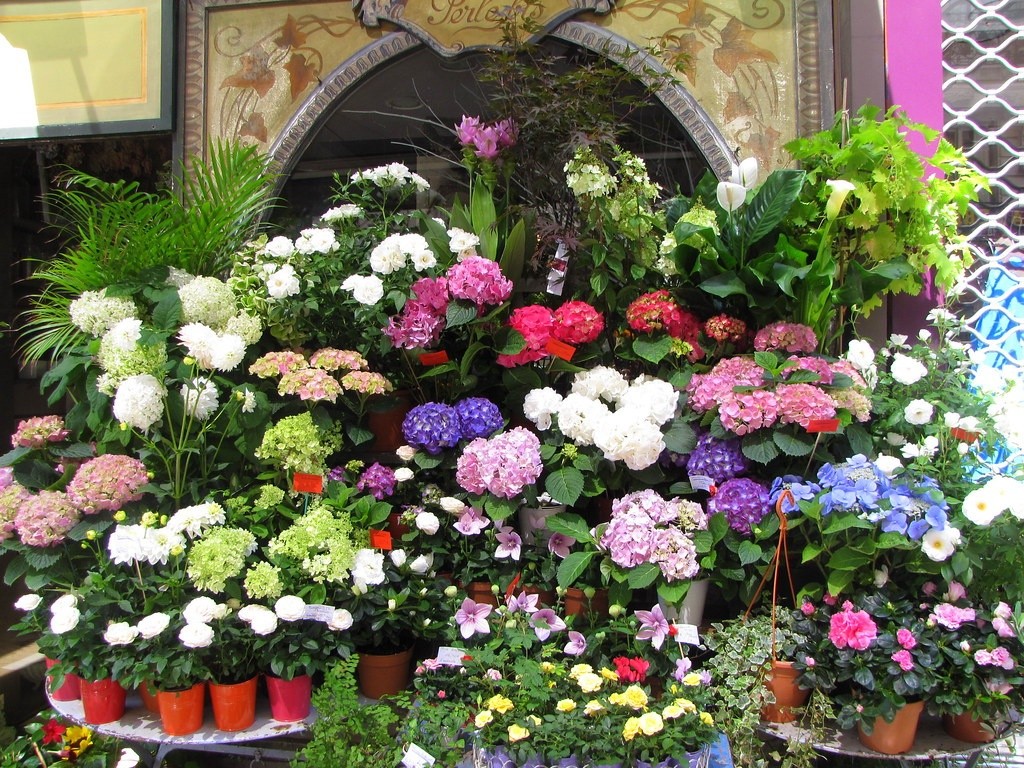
0 3 1024 768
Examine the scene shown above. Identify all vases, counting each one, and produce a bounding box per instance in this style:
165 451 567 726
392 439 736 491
755 658 809 723
357 640 414 702
153 679 206 736
77 671 127 724
943 703 998 743
564 586 609 616
140 679 160 713
367 389 409 451
659 572 711 626
207 672 259 730
513 583 554 611
45 655 78 701
468 582 498 613
519 504 567 556
598 497 614 524
266 670 312 722
852 683 926 755
